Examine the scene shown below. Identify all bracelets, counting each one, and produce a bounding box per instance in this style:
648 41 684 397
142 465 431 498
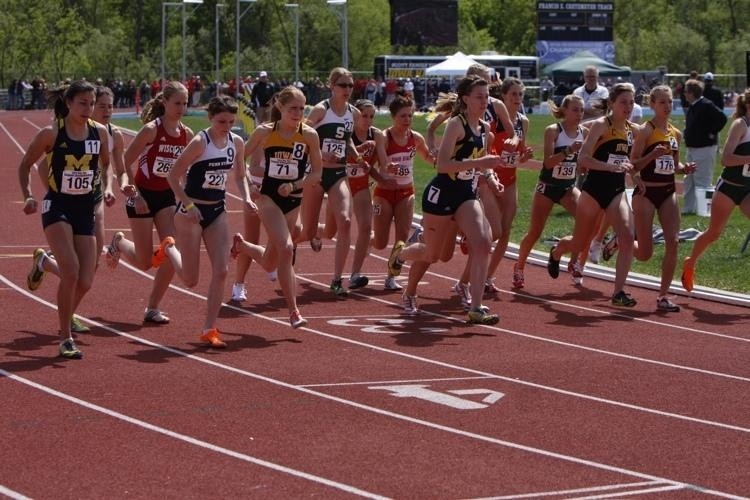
288 182 297 193
23 196 34 203
484 171 496 180
183 203 196 210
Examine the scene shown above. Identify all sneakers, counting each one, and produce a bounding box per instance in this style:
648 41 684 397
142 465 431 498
106 232 124 273
143 305 170 324
547 245 561 279
454 278 500 325
230 232 244 264
681 256 695 292
152 236 176 268
512 262 525 288
568 260 585 286
591 236 619 264
328 274 368 297
611 291 637 307
385 226 426 316
267 248 296 281
289 309 308 328
232 281 247 301
657 297 680 312
26 248 55 291
199 327 227 348
311 238 323 253
59 317 92 359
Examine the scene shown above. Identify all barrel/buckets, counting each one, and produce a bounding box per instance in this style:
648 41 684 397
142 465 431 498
625 189 634 211
695 186 716 218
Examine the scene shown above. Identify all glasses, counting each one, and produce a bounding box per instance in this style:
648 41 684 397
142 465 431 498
331 82 354 89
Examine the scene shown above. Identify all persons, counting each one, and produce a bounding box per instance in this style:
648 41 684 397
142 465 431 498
16 81 117 362
387 75 506 325
106 81 196 325
7 67 750 317
152 94 261 351
228 85 325 329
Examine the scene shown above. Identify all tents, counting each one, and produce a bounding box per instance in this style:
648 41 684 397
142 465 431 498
537 51 631 100
424 50 500 107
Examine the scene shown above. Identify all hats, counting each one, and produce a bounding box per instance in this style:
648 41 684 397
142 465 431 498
260 70 267 76
703 71 715 81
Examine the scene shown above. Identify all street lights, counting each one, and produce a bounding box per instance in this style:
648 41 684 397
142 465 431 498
153 1 355 107
